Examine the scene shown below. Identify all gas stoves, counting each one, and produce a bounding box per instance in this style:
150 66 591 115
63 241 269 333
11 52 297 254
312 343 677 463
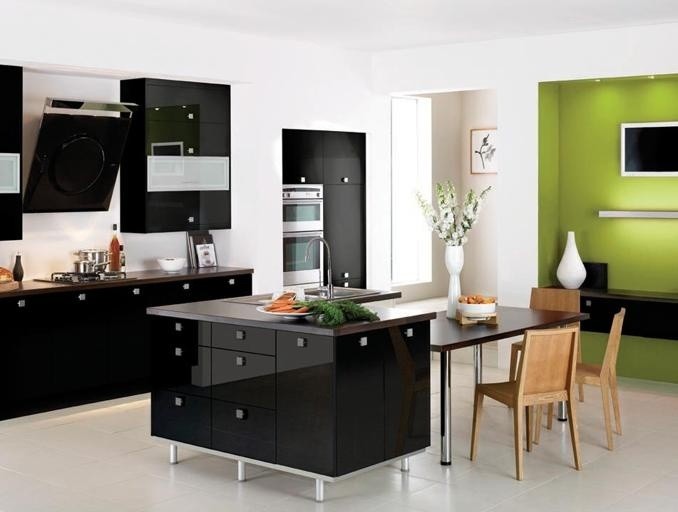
34 273 137 287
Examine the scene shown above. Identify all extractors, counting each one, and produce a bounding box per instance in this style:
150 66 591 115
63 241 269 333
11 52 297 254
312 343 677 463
22 98 140 211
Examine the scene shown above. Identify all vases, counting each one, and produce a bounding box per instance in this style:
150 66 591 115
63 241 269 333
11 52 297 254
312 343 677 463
445 239 466 318
553 229 590 292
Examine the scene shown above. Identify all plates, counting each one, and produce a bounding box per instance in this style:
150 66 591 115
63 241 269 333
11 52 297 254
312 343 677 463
258 302 325 318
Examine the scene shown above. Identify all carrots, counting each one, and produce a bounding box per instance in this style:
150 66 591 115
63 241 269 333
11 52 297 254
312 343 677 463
266 300 309 313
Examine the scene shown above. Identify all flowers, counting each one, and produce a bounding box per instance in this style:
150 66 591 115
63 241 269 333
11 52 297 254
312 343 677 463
408 176 494 246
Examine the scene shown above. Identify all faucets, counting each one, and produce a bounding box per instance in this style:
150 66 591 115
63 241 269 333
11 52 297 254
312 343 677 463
302 235 333 300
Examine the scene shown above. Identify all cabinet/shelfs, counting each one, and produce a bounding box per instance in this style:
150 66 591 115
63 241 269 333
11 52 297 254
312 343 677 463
147 306 213 466
209 315 278 484
112 276 172 409
322 129 367 187
0 287 53 428
119 79 235 231
282 128 324 185
164 271 210 305
323 184 367 280
49 280 117 421
210 267 255 300
386 311 440 474
0 65 27 242
271 320 386 502
323 278 367 291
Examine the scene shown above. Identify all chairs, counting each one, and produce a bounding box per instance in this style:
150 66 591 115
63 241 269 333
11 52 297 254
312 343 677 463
533 306 630 450
466 326 588 482
506 284 586 429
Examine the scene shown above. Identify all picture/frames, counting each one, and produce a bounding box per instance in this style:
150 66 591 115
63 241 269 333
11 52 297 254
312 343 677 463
467 126 501 178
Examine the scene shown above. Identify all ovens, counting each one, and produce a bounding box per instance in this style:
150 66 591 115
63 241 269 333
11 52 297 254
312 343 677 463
282 184 323 285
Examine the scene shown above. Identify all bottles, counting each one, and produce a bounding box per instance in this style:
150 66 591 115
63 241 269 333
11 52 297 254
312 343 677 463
110 224 125 272
13 250 24 283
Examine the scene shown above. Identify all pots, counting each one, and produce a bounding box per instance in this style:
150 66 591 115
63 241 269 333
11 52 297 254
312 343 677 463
72 248 109 274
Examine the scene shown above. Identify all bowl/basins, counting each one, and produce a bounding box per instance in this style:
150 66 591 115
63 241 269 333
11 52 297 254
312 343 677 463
458 302 496 314
157 257 186 275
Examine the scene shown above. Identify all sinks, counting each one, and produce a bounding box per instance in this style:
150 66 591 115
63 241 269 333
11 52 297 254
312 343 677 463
303 284 365 299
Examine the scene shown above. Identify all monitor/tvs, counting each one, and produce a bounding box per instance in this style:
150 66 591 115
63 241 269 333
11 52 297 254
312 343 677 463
621 122 678 177
